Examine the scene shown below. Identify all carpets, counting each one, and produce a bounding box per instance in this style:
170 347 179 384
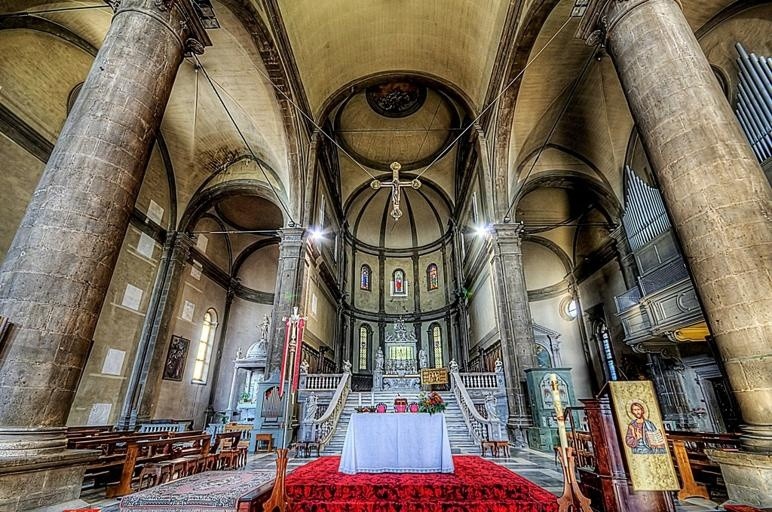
119 468 289 512
279 454 560 512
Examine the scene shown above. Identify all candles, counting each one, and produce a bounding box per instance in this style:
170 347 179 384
549 374 569 468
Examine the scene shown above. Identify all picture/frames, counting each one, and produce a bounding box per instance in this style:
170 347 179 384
162 334 191 383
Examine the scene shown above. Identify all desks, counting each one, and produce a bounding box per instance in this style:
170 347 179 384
337 412 456 476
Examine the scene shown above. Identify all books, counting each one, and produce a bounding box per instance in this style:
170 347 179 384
644 427 665 448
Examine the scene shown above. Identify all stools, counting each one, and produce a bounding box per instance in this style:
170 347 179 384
293 441 321 459
65 411 249 499
481 440 511 458
553 427 598 467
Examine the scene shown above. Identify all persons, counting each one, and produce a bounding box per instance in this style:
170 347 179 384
341 358 353 376
624 402 668 455
303 391 320 421
362 267 368 287
393 315 407 340
494 357 503 373
374 346 385 370
299 358 310 375
256 312 271 340
396 274 401 291
429 269 436 289
417 346 430 369
381 178 412 205
448 357 459 373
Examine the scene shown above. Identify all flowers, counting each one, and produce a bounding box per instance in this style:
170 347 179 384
417 390 450 416
